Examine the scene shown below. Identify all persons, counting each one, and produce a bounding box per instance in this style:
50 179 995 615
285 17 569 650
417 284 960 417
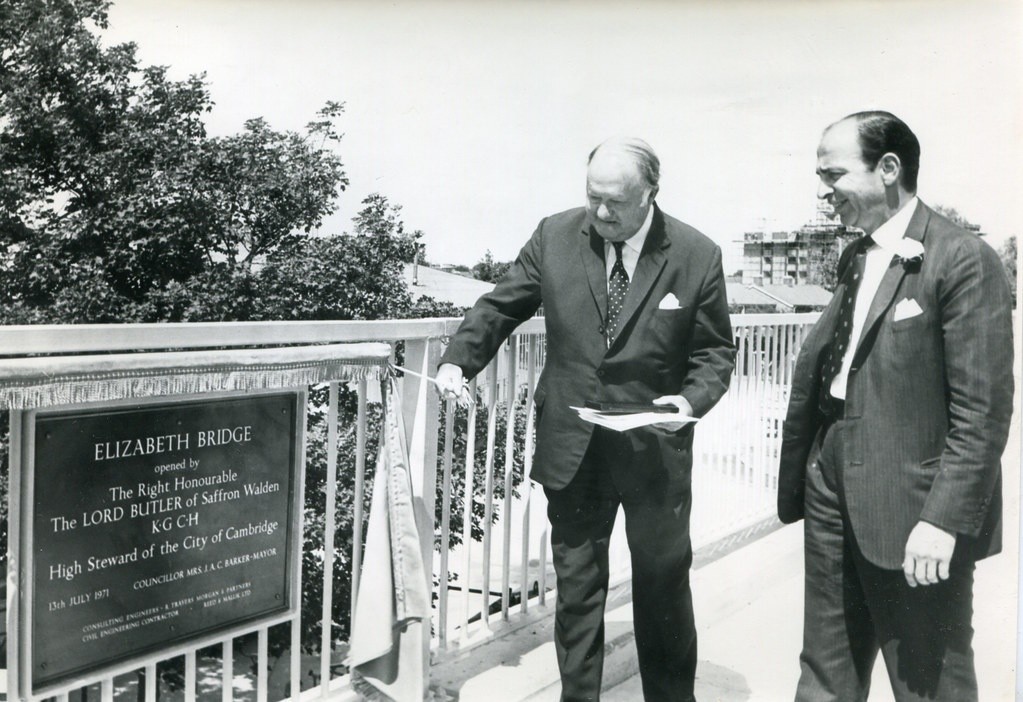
433 136 740 702
777 111 1016 702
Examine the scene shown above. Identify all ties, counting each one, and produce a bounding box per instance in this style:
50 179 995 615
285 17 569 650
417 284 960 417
607 242 630 347
819 240 868 416
